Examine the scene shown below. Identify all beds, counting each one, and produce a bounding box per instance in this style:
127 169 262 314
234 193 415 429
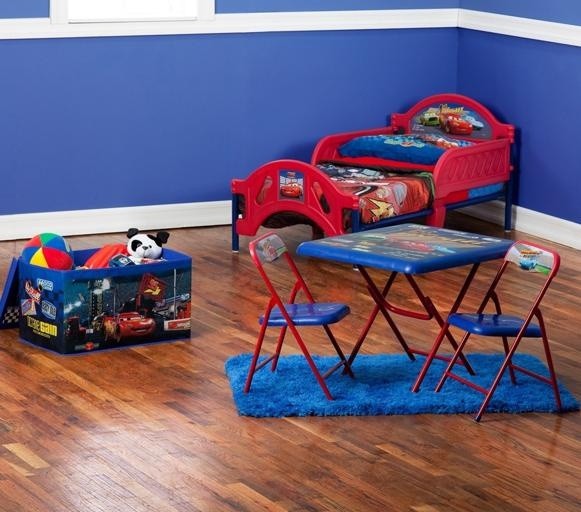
229 92 520 272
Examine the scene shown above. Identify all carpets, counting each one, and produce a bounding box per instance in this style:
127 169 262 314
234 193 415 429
223 351 578 419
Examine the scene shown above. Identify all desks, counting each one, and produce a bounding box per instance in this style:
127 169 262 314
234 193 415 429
295 220 518 394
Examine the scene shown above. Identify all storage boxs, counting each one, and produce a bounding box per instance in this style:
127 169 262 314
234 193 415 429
0 242 192 356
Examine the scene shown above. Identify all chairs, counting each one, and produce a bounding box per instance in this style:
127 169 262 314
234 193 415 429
409 236 562 422
246 232 357 401
